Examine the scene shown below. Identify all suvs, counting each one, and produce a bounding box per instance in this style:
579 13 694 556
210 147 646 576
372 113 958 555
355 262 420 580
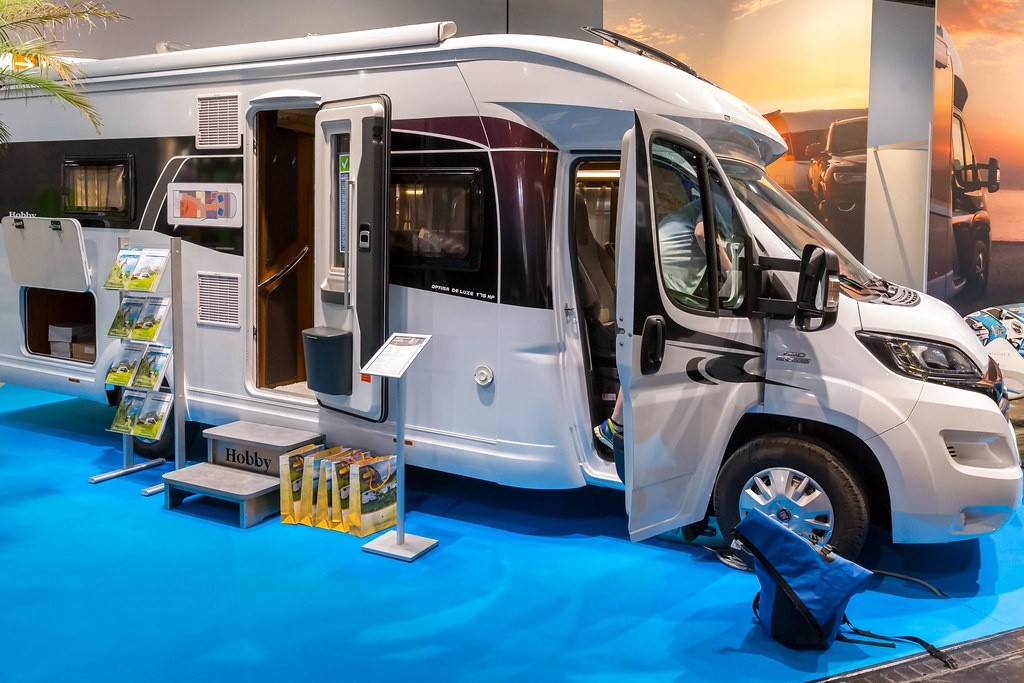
807 104 1005 296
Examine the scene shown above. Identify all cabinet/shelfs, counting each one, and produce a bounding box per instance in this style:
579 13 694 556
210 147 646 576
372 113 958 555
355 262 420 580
89 234 187 497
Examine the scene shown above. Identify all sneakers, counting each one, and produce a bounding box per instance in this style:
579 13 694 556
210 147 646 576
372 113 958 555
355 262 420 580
593 418 617 450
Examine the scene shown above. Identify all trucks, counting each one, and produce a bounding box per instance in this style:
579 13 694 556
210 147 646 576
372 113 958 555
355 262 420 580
0 19 1024 569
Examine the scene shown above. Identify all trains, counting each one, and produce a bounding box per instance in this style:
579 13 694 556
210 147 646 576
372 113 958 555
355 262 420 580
292 478 304 492
359 481 397 507
338 485 350 499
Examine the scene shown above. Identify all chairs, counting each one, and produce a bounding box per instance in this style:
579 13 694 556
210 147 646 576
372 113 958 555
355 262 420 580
573 193 619 371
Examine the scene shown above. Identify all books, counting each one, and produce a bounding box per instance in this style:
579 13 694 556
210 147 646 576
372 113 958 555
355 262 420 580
104 248 174 440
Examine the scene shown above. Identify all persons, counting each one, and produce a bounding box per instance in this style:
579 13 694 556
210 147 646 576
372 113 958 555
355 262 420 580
593 177 748 453
